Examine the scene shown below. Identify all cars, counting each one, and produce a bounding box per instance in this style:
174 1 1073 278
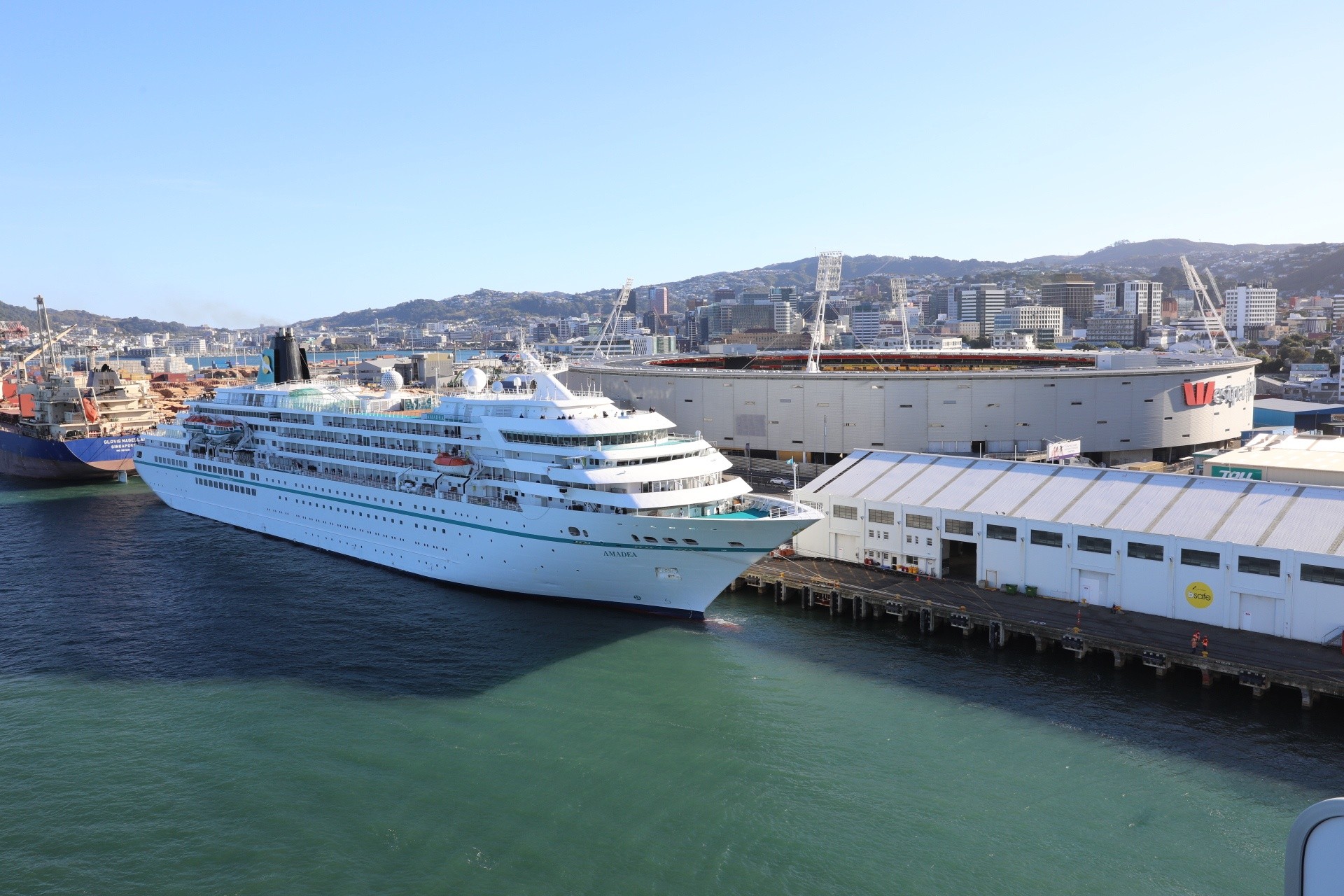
770 477 791 486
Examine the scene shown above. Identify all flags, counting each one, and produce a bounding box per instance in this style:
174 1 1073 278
787 458 793 464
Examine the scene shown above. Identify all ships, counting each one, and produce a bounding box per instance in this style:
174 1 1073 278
131 325 826 621
0 295 167 482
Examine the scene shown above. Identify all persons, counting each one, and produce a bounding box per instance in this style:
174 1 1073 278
1052 446 1063 457
716 501 720 514
679 508 684 518
615 507 627 515
520 404 656 419
369 423 456 466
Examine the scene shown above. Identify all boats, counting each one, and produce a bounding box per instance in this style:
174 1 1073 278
432 452 472 476
82 398 97 424
204 421 244 444
183 416 213 434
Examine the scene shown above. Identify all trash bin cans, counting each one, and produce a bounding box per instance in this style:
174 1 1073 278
1025 585 1037 597
1006 584 1018 595
